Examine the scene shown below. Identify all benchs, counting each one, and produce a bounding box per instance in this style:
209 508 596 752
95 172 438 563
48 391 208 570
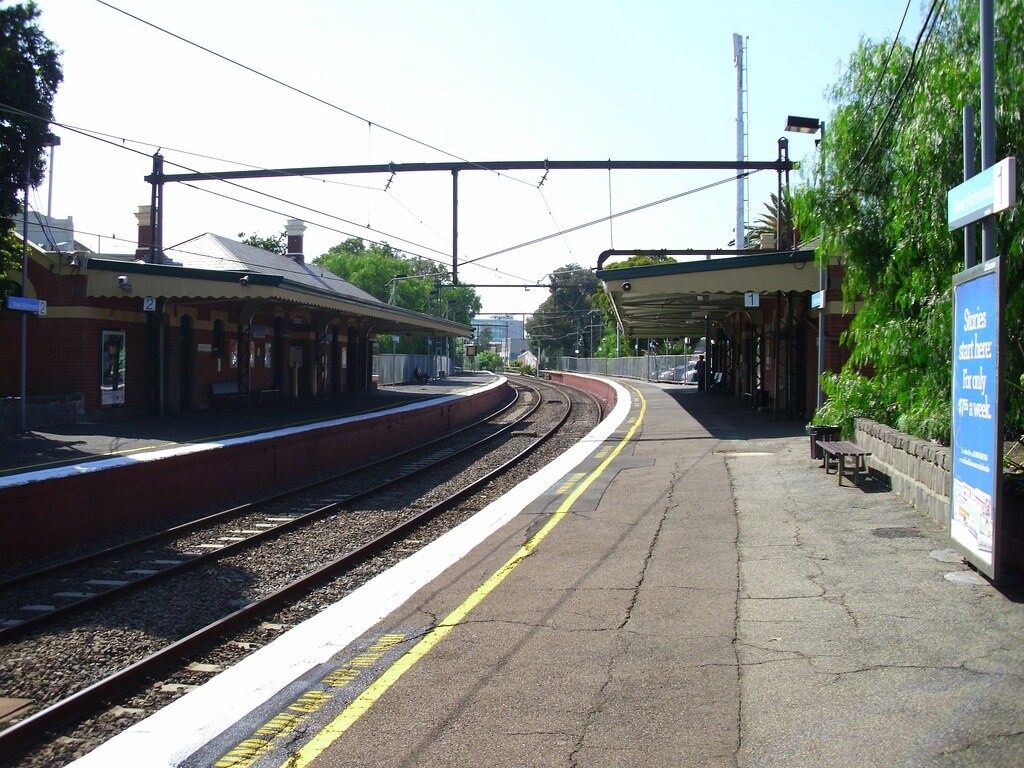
816 439 870 486
212 384 252 407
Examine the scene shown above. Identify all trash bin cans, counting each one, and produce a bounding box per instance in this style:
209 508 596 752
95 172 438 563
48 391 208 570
439 371 446 379
752 389 769 405
810 425 842 459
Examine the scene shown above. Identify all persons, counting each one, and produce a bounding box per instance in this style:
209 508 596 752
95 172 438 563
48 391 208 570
694 355 705 393
104 335 122 391
414 365 433 385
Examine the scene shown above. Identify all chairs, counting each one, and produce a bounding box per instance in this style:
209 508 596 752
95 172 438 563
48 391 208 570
708 372 722 391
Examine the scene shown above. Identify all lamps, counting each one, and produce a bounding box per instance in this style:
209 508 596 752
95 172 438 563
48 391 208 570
71 256 78 268
239 276 249 286
622 281 632 291
